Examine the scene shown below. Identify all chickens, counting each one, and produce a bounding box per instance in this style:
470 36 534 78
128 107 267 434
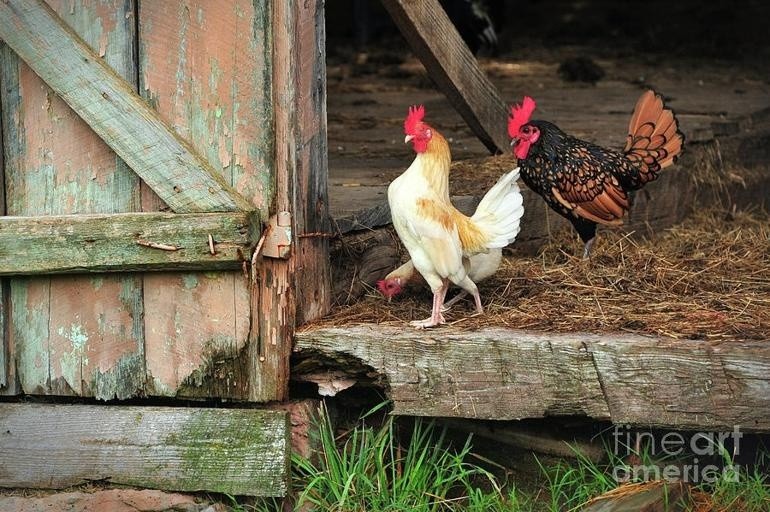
507 87 685 258
377 248 503 311
387 103 525 330
